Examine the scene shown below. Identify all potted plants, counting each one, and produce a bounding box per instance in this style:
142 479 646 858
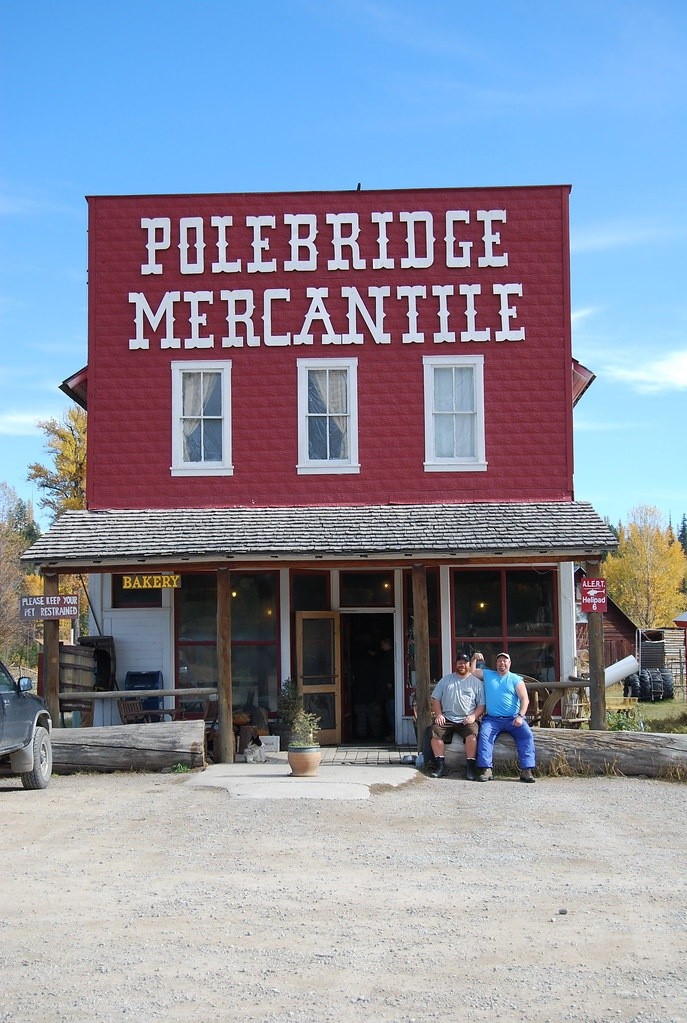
276 677 310 750
288 708 321 777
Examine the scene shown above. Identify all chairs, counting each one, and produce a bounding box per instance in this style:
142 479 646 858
117 698 186 725
203 699 219 763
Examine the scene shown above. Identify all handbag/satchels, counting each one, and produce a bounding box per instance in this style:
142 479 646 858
374 680 394 700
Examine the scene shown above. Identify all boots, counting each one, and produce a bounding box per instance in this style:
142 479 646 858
467 759 478 779
430 756 448 778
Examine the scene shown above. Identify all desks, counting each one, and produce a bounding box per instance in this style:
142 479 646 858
123 709 176 724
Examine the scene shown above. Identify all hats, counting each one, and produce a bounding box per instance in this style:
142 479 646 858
456 653 471 662
496 653 510 660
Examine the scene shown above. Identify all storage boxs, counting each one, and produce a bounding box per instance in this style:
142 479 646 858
237 736 280 753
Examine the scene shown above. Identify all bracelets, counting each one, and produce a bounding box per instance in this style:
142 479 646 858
518 713 523 719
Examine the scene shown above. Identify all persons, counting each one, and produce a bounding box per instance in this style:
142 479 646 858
431 651 486 782
470 653 536 783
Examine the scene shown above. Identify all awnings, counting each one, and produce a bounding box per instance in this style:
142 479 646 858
18 506 621 567
58 365 88 411
572 358 597 409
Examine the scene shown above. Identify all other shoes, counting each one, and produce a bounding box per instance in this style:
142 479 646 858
521 767 535 782
478 767 494 781
359 735 367 741
385 735 394 743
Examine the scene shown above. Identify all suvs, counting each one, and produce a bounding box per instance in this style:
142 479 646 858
0 662 52 789
